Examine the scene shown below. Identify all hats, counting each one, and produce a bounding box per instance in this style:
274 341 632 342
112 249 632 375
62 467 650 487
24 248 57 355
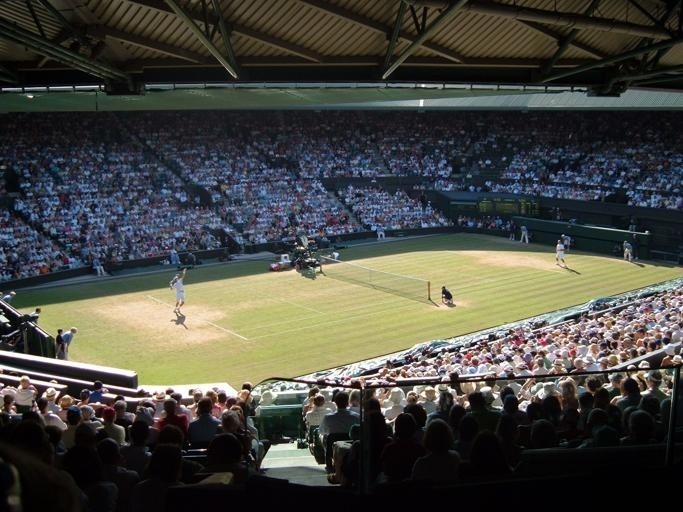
389 347 683 401
42 388 278 424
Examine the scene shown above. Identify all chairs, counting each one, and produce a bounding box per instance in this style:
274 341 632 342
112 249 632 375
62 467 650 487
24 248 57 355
0 377 682 511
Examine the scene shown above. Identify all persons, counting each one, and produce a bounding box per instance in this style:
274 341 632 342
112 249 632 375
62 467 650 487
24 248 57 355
1 107 682 510
